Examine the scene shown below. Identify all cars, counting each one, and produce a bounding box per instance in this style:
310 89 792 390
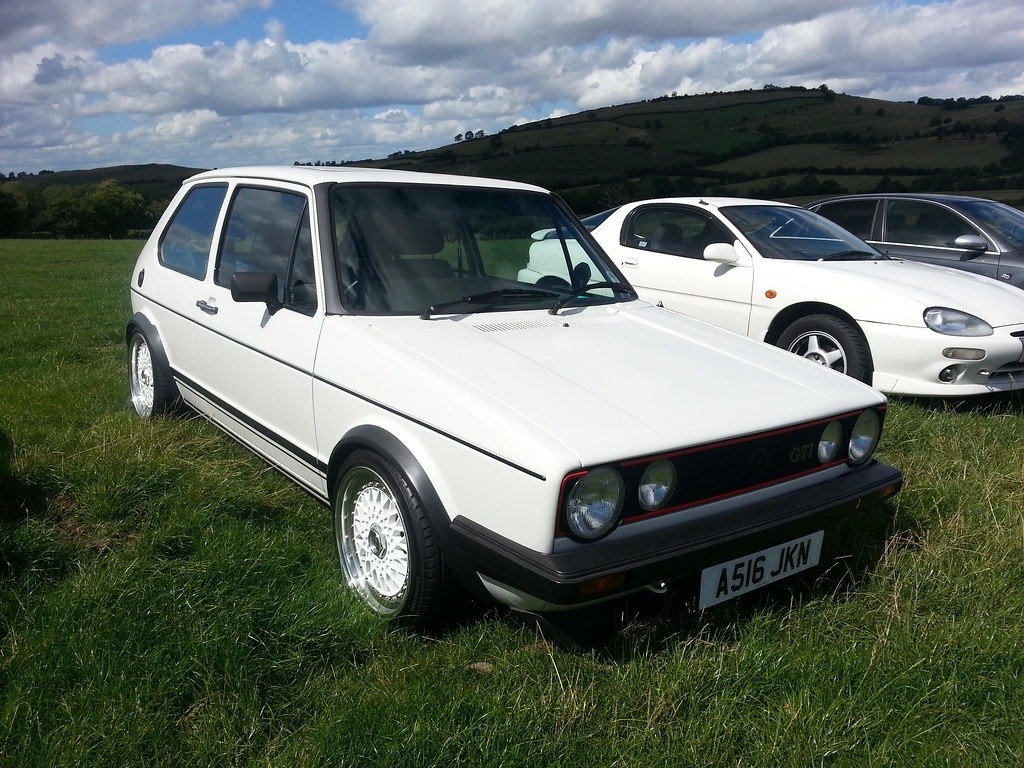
126 168 904 640
517 196 1024 398
742 193 1024 290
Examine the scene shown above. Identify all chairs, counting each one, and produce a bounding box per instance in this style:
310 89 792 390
359 201 464 311
651 221 684 255
